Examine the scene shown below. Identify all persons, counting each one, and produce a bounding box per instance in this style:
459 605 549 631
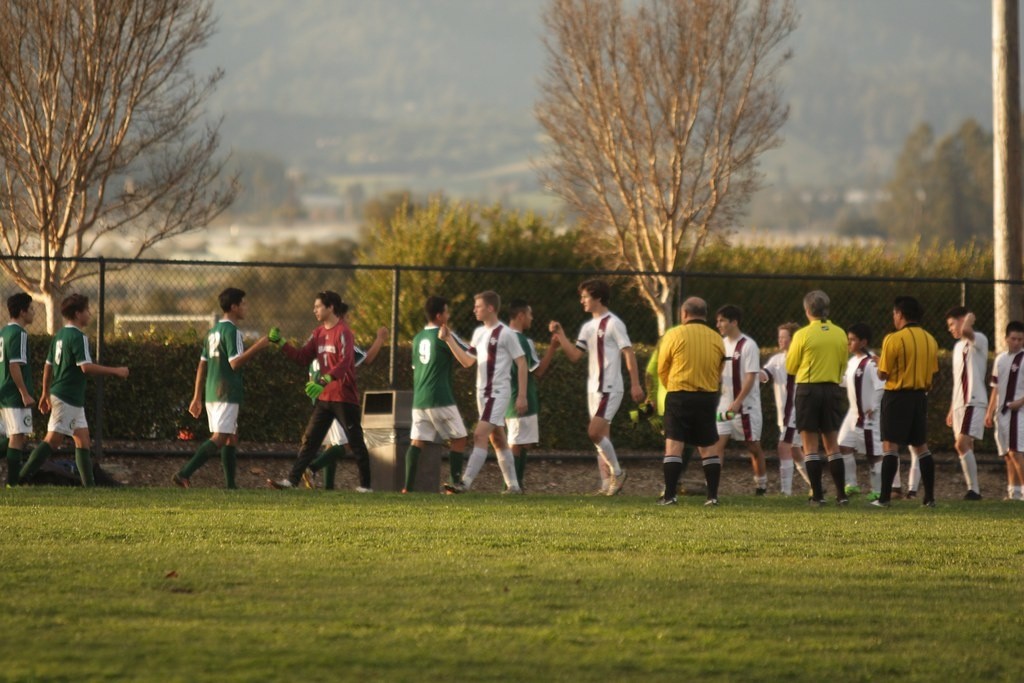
266 290 389 492
760 321 920 500
645 296 768 507
0 291 39 487
785 290 850 505
503 280 643 496
870 296 939 508
983 321 1024 502
945 307 987 500
401 291 528 495
19 294 129 488
171 288 269 488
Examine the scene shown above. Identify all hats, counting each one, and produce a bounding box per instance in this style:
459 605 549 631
628 403 654 424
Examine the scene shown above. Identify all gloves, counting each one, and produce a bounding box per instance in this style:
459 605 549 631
305 373 331 400
269 327 286 349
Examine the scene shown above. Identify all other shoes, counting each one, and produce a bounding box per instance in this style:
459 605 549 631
593 468 629 497
962 489 983 500
835 484 861 505
865 490 881 501
501 488 523 495
444 482 468 494
1003 489 1024 501
870 499 888 510
302 464 316 490
4 475 24 487
807 486 827 504
172 473 193 490
265 478 298 491
655 495 678 507
889 491 915 500
356 486 373 493
755 488 767 496
704 499 719 506
919 499 935 507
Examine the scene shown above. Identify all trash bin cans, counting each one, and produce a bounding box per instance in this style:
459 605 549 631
361 391 442 491
